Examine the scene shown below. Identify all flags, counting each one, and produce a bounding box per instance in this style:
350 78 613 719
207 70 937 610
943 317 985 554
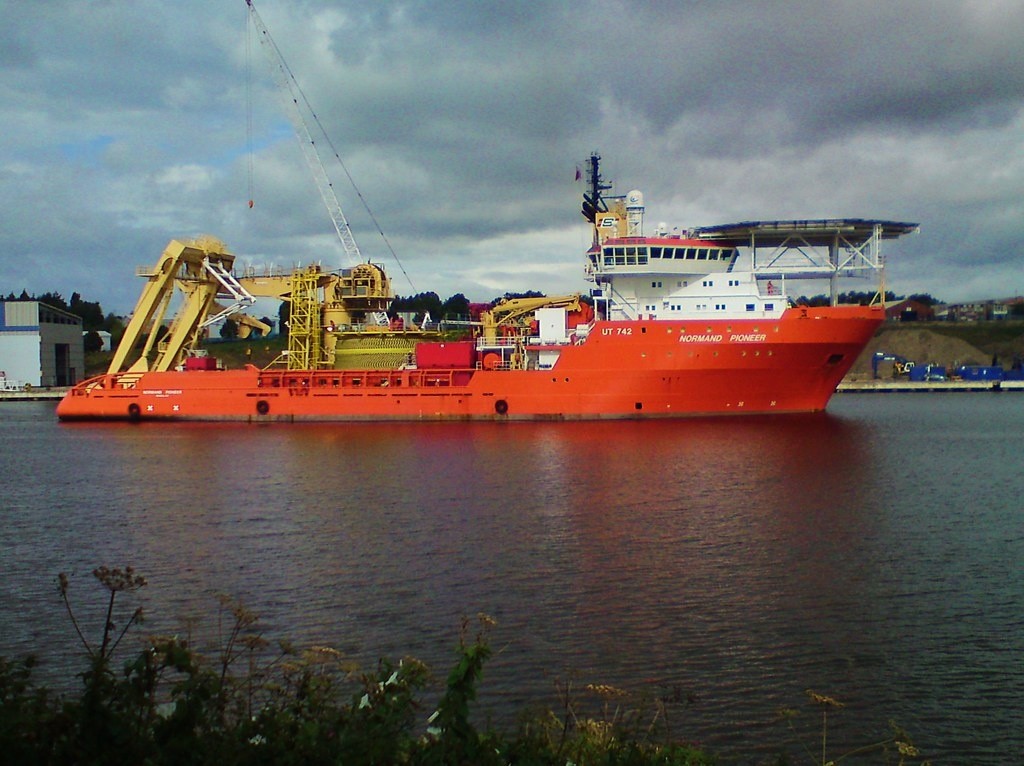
575 166 582 181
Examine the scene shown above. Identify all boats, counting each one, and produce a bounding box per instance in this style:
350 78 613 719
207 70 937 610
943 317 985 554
57 153 886 420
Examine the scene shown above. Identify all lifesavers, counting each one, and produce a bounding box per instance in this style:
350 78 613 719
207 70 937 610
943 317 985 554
128 403 140 417
256 400 269 414
495 400 508 414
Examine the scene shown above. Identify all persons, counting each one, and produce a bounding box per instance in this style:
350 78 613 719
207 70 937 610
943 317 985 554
767 281 773 296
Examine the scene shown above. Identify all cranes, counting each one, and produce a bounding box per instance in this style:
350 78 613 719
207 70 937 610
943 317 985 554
246 0 418 296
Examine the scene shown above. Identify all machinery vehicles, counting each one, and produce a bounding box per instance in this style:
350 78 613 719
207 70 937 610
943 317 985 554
872 352 914 379
